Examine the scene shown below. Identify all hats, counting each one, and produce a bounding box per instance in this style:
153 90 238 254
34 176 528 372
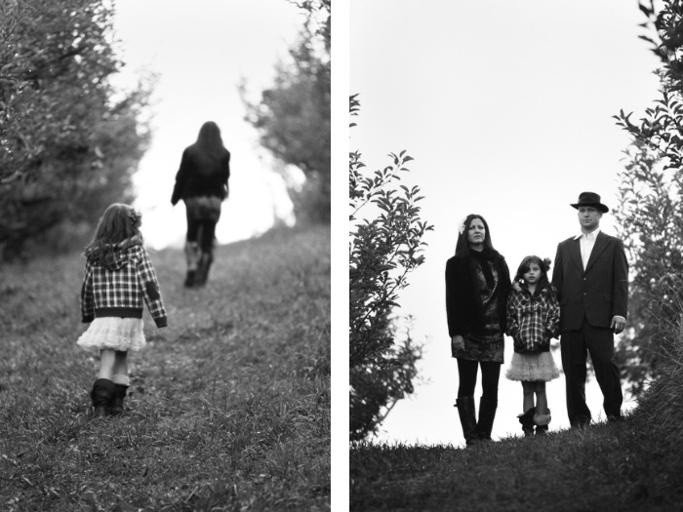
570 192 608 213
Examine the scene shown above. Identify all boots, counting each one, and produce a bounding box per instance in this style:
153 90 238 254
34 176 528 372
106 382 128 415
184 242 197 286
535 409 551 435
197 248 212 286
91 379 114 405
477 396 497 442
454 397 478 446
517 407 537 437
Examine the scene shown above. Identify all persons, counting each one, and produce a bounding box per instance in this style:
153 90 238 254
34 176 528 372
550 192 628 430
504 255 561 436
169 120 231 287
76 202 167 418
443 213 511 445
